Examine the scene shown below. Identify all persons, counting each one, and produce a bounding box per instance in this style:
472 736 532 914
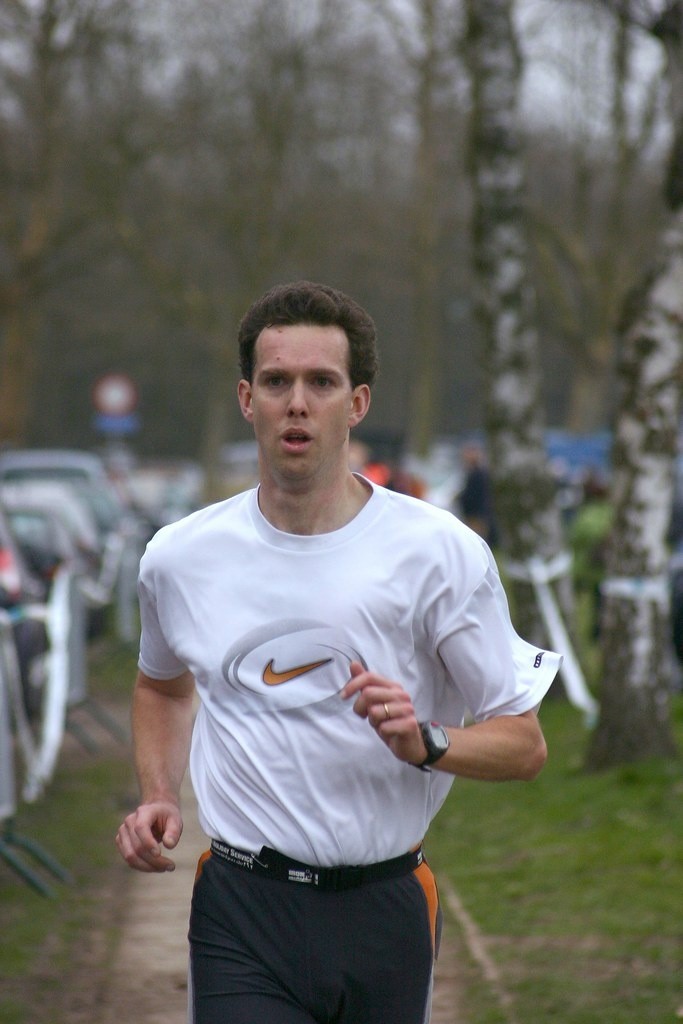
343 427 500 552
116 280 563 1023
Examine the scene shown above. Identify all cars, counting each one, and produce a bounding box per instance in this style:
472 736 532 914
0 445 206 735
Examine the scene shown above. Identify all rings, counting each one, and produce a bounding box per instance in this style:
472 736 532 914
381 700 390 721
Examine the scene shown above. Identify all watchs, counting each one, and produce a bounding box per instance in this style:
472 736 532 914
407 720 449 772
